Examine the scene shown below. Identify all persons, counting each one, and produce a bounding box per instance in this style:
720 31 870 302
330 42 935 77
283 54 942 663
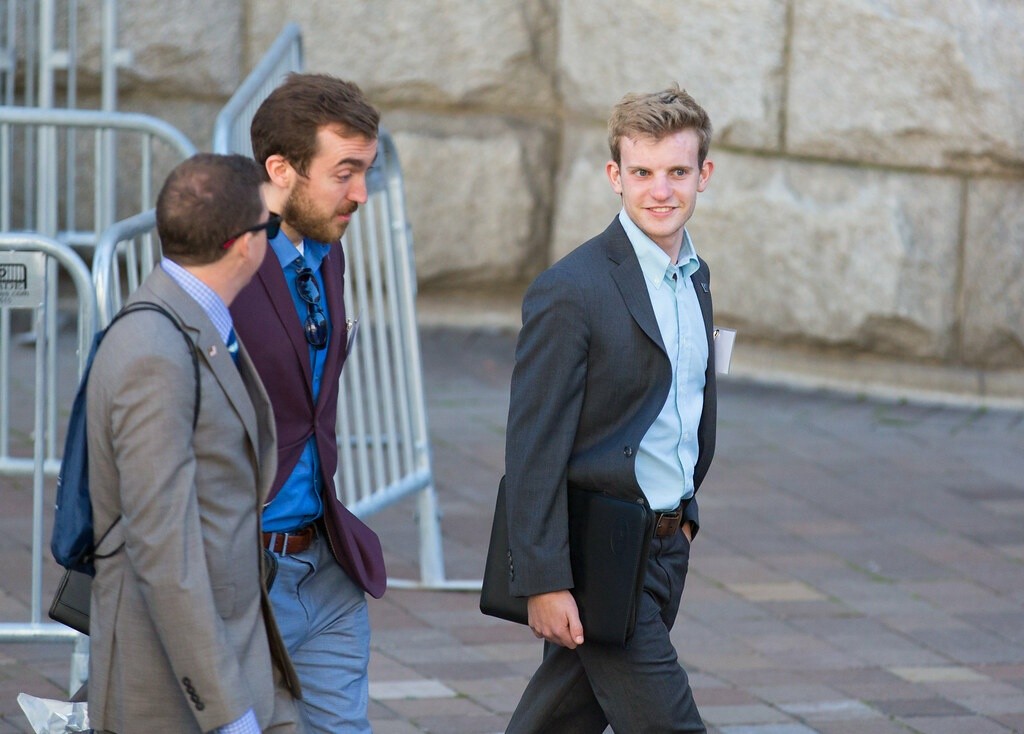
88 154 300 734
501 81 735 734
226 72 386 734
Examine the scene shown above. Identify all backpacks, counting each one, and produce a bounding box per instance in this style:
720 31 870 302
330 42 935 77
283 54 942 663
50 302 200 577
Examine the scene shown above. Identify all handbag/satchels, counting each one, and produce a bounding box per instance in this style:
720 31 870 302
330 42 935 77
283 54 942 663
479 474 647 648
48 542 277 638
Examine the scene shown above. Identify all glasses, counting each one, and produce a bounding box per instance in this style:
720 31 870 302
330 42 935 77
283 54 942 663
223 212 283 248
295 267 328 350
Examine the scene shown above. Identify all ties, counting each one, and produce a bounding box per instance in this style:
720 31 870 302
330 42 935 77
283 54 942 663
224 328 239 367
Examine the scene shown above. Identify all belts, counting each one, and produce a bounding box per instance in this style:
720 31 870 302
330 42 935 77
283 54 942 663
261 520 323 555
651 500 683 537
